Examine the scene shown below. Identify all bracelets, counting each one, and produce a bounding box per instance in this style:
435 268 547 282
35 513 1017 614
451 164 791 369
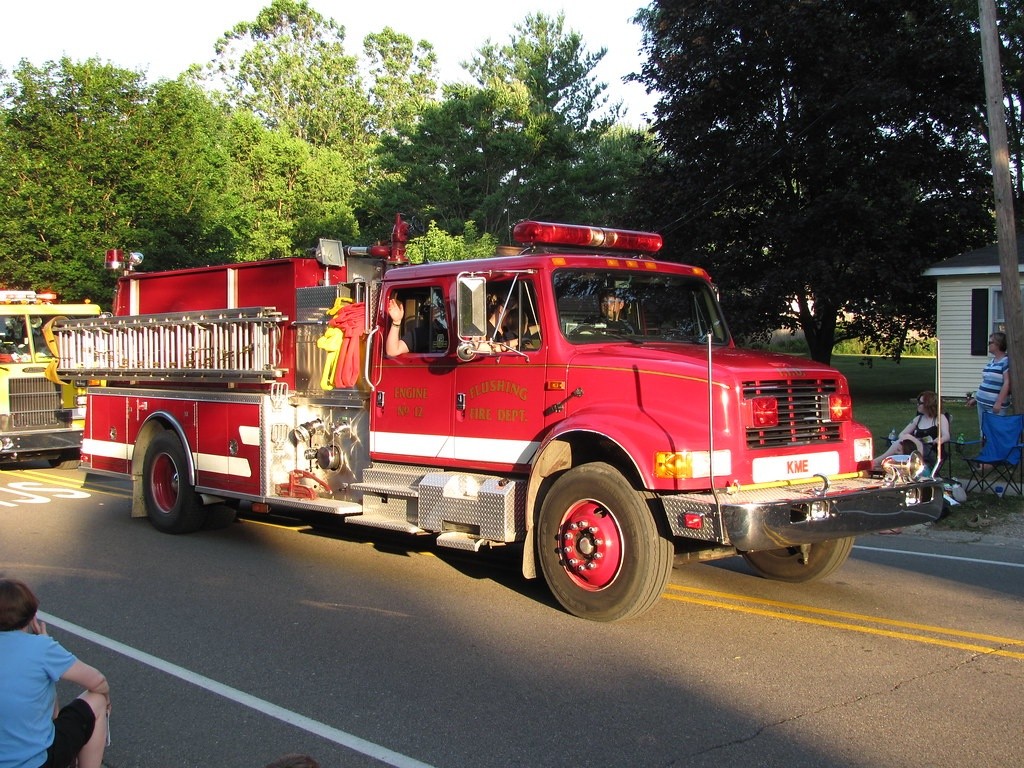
392 322 401 326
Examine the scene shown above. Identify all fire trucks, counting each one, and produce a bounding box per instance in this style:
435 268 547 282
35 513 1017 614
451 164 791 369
41 220 943 620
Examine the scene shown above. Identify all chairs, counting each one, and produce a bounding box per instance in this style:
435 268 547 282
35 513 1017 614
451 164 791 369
951 411 1024 500
881 411 951 478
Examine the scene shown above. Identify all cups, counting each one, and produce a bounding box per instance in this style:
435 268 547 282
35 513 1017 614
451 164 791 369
996 486 1003 496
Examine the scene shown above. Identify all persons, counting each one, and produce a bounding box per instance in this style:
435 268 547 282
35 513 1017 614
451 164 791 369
0 578 112 768
967 333 1010 467
386 294 447 356
584 288 641 335
480 292 533 351
874 391 950 534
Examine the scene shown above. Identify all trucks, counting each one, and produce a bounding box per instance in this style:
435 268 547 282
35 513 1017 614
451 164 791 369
0 289 113 469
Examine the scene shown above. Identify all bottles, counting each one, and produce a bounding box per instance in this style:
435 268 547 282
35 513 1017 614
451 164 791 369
955 433 966 453
888 429 897 447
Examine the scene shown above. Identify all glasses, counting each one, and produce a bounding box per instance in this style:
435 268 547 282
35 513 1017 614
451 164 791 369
989 342 995 345
918 400 924 405
